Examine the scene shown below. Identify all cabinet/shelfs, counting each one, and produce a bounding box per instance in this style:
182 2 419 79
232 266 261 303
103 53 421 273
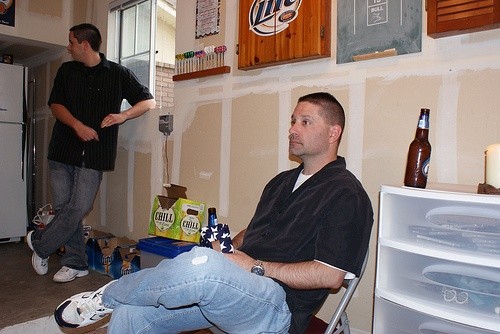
372 186 500 334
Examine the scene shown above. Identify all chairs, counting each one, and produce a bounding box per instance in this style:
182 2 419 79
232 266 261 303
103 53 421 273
301 247 369 334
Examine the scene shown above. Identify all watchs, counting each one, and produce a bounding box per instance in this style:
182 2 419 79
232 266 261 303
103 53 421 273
250 259 265 277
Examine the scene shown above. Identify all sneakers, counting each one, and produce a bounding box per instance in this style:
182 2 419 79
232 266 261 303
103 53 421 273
53 265 89 283
53 279 119 328
26 230 50 275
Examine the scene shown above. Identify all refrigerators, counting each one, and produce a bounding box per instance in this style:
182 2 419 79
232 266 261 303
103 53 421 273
0 63 29 244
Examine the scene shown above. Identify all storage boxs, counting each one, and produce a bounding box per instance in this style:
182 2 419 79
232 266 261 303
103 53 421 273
81 184 206 280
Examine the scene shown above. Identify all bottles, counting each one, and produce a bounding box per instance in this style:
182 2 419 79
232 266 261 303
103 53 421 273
404 108 431 189
208 208 218 226
38 209 55 230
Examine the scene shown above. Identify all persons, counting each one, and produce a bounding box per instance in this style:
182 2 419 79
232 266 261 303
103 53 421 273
26 23 156 283
54 92 374 334
